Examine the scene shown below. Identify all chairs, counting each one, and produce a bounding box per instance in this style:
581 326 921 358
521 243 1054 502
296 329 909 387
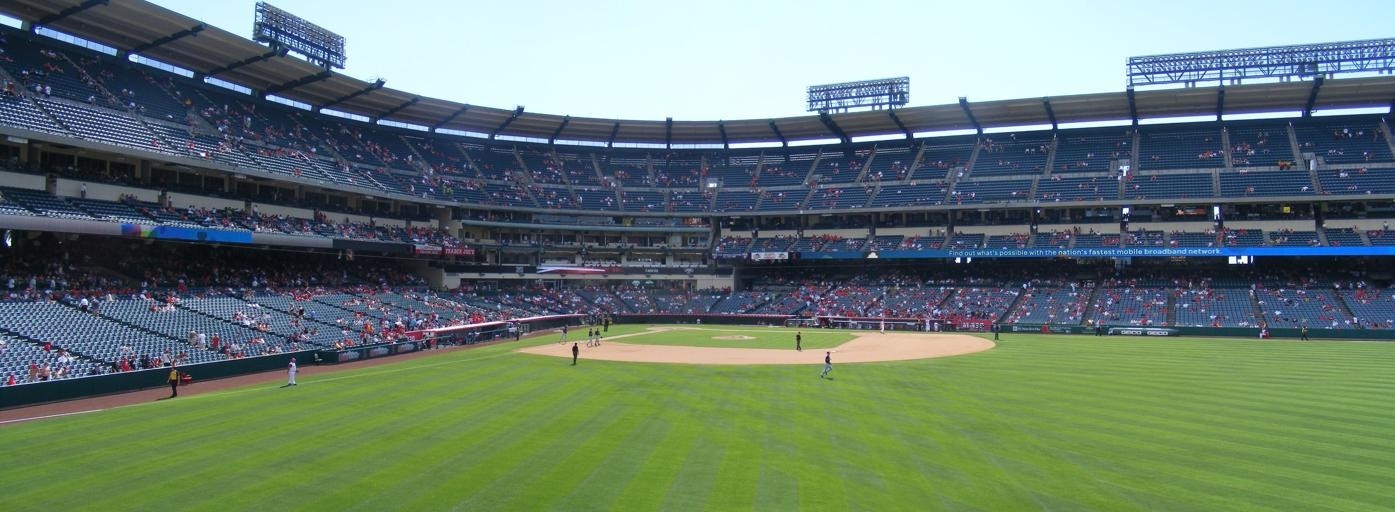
0 91 394 191
1 280 379 387
0 186 251 233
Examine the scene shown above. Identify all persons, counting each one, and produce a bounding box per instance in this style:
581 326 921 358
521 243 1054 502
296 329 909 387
0 31 1395 398
